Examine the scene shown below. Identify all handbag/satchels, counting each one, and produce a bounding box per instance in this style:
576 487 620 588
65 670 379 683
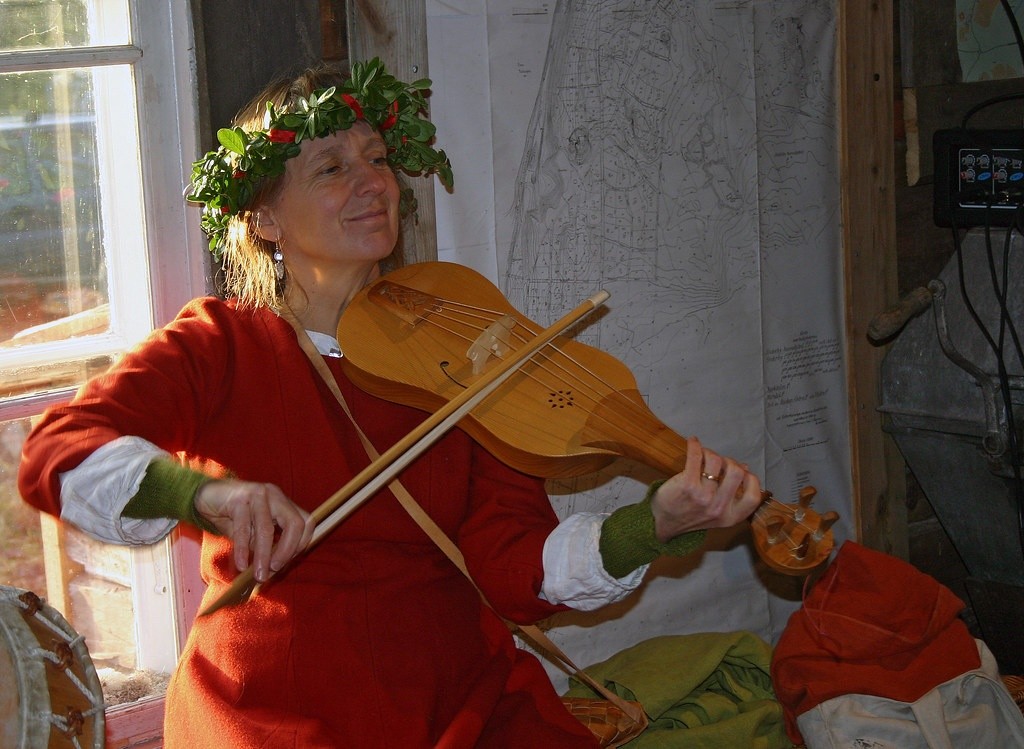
795 638 1023 749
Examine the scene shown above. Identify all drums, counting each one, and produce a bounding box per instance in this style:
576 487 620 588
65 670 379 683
0 586 106 749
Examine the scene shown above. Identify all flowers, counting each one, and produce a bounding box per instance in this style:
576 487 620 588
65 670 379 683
183 56 454 262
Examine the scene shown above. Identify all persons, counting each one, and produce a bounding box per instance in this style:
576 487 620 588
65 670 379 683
18 71 761 748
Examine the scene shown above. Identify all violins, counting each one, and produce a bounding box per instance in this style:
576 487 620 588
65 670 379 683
336 262 840 576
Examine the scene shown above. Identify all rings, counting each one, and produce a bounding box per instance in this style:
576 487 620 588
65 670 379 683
700 472 719 481
